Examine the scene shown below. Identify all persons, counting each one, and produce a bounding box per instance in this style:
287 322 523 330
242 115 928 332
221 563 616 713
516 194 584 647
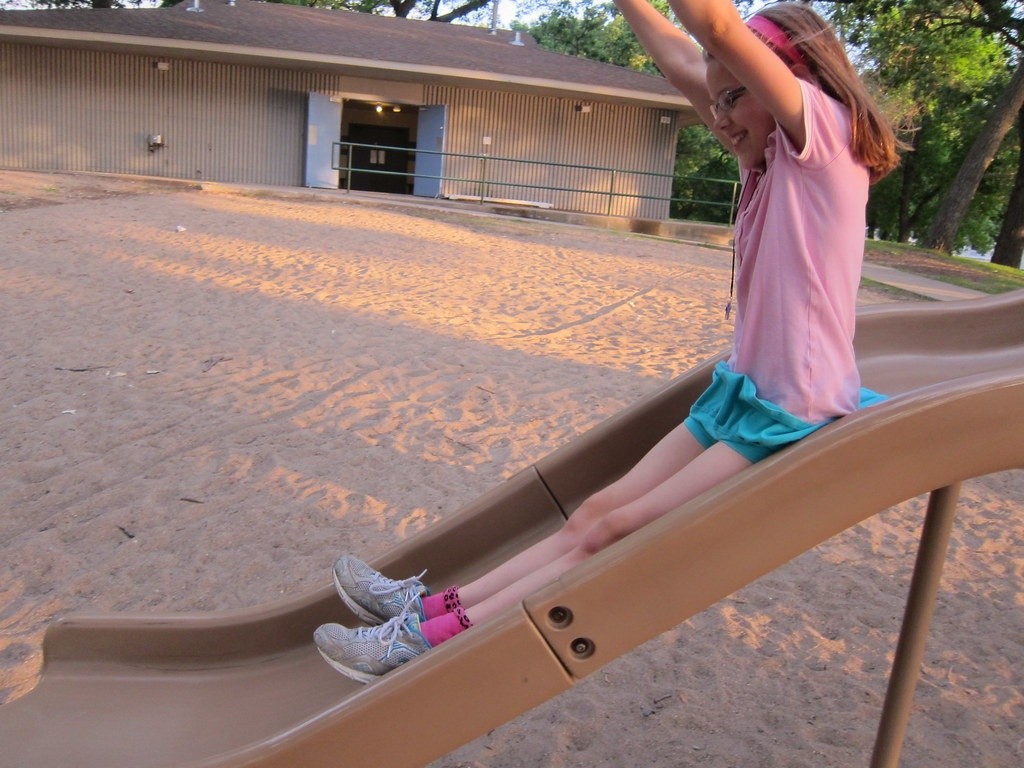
313 0 923 684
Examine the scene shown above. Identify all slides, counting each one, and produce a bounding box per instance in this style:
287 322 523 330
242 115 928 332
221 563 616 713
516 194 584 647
1 287 1024 767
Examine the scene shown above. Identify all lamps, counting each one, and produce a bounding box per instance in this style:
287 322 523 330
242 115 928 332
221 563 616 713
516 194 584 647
375 103 383 112
392 104 400 112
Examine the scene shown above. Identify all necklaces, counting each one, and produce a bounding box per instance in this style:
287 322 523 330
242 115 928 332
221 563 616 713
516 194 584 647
723 165 767 320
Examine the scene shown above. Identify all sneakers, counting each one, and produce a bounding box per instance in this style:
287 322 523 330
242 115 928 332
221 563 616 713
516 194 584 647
332 555 431 628
312 592 433 684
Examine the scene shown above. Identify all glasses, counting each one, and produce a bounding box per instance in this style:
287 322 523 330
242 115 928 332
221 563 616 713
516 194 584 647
710 85 746 119
